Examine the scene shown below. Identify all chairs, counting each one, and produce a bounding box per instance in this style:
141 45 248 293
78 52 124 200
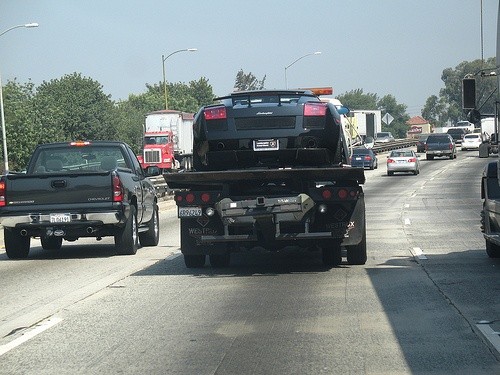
101 157 116 170
46 160 63 172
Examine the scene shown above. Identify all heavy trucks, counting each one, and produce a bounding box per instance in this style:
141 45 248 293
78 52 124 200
136 110 193 177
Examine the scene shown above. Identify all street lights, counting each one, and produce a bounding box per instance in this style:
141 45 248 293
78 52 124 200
0 22 40 172
284 51 322 90
162 48 198 110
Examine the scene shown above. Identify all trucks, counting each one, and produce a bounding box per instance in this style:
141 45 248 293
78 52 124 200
162 87 375 269
456 120 475 134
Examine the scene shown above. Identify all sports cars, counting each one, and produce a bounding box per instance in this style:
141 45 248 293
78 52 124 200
192 90 351 171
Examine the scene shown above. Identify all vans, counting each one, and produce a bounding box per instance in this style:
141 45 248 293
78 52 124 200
424 133 457 160
446 128 466 143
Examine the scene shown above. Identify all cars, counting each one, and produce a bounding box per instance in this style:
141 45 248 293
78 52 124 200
416 133 430 152
461 133 482 151
386 150 421 176
375 132 397 143
349 149 378 170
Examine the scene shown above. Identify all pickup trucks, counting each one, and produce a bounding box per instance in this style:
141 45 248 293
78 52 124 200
0 140 161 260
